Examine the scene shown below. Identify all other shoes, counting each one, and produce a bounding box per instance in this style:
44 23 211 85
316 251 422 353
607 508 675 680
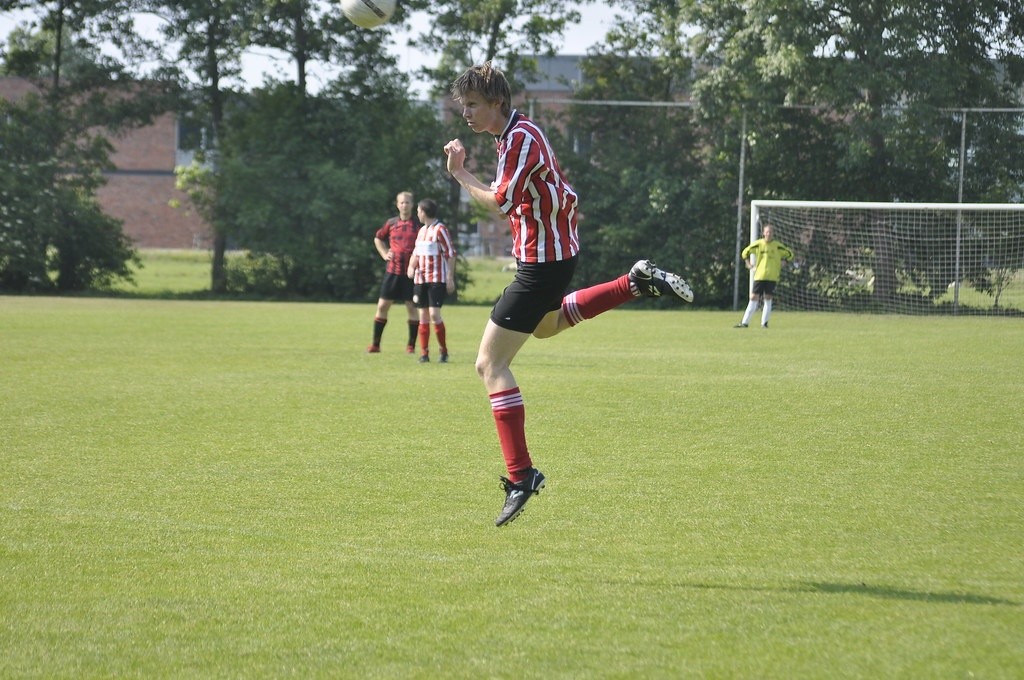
407 346 414 352
761 321 768 328
368 346 379 352
734 323 748 328
417 355 429 362
438 352 448 362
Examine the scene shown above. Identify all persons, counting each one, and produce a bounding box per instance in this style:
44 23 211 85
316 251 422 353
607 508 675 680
368 191 457 364
444 64 694 527
734 223 794 328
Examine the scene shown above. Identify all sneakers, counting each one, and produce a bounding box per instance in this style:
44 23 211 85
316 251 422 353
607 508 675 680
494 466 546 526
628 258 694 304
